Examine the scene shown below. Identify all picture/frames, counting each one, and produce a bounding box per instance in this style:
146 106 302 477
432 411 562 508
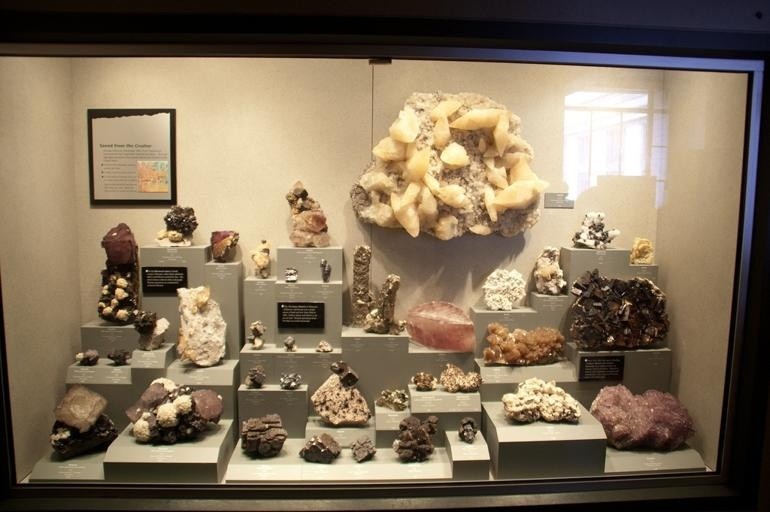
87 108 176 204
